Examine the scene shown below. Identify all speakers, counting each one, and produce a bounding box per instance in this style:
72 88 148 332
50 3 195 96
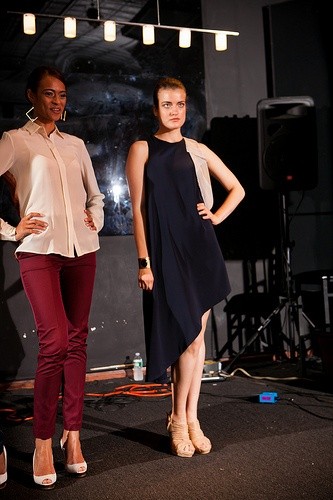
257 96 319 191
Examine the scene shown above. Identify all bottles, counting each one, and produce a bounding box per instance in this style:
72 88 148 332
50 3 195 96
133 353 143 381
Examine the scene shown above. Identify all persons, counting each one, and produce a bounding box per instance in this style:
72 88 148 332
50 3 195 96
125 77 246 457
0 66 104 485
0 441 8 485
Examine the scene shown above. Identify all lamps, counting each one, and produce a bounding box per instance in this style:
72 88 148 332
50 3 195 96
64 17 77 39
103 20 118 43
142 24 156 45
215 33 228 52
23 13 36 35
178 29 192 48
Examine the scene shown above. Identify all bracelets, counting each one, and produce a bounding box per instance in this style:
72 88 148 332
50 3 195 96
138 257 150 269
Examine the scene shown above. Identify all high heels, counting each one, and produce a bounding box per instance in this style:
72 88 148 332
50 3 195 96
33 448 58 486
0 446 8 489
61 438 87 474
167 414 195 459
188 421 213 454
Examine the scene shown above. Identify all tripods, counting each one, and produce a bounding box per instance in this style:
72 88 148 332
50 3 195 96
221 190 333 377
